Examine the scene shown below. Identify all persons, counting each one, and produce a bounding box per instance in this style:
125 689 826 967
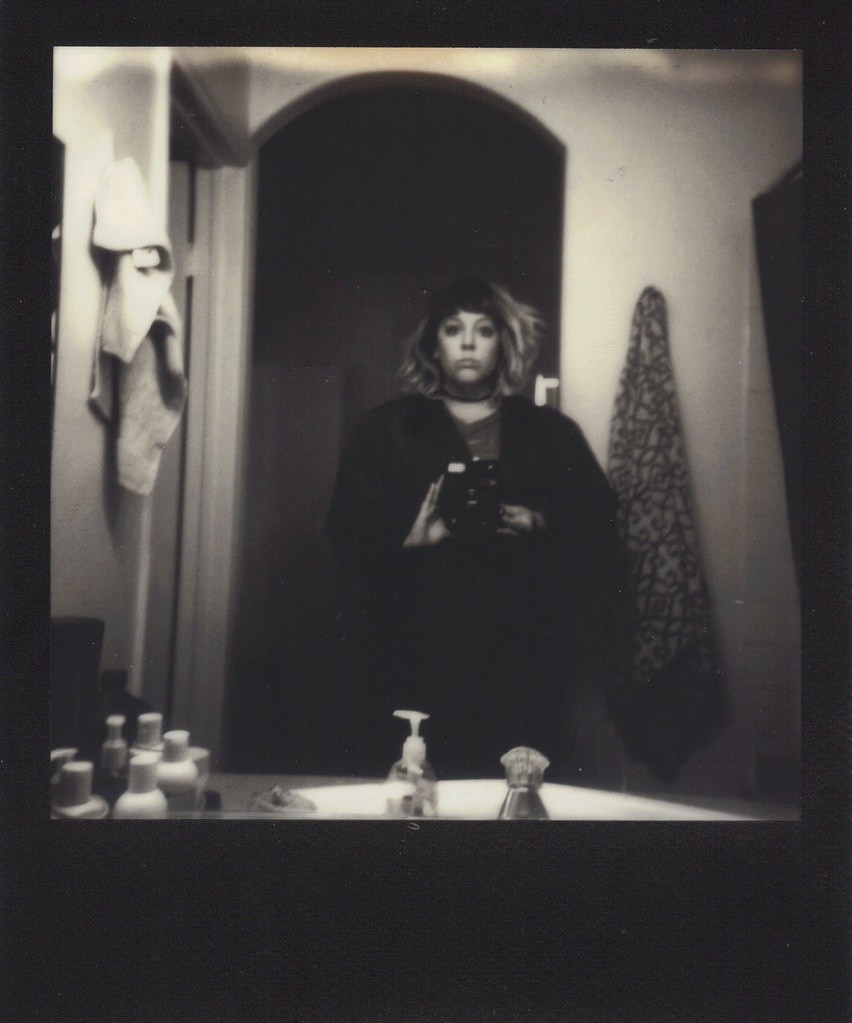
327 282 617 782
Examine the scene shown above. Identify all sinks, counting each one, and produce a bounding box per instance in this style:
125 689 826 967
276 772 753 820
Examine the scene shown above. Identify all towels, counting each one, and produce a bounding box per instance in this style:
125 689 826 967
599 284 727 771
86 156 191 495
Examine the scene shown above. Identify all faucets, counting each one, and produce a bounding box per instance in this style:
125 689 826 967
496 746 552 820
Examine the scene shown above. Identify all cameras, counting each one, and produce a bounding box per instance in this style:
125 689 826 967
438 438 503 525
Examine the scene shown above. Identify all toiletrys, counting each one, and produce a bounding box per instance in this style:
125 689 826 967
51 614 214 822
388 708 439 819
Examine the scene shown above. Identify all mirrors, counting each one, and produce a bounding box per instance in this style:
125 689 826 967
46 35 817 830
